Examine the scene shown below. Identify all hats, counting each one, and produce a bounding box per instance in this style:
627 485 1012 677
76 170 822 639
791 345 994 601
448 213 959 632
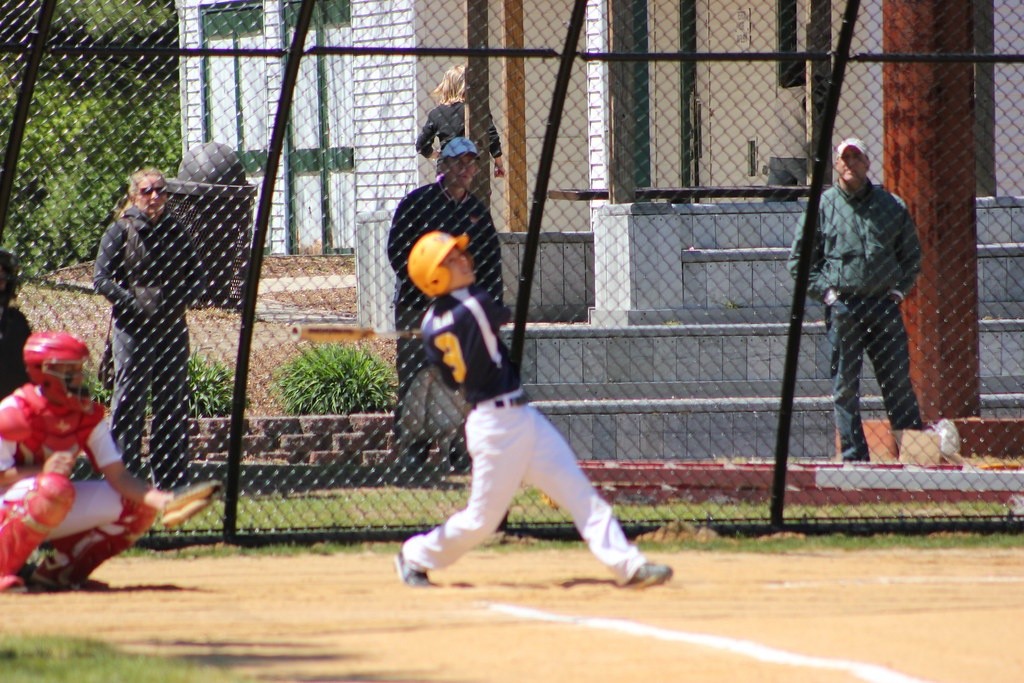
837 137 869 155
437 137 482 169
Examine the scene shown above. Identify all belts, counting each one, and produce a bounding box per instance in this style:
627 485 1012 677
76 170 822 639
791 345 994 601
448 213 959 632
472 391 529 410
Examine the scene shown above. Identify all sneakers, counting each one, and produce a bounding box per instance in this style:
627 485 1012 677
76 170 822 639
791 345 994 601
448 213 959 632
0 575 27 593
28 569 109 593
394 551 434 587
626 562 672 588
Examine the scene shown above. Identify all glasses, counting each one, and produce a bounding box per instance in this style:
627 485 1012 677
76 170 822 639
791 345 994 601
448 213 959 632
139 187 169 196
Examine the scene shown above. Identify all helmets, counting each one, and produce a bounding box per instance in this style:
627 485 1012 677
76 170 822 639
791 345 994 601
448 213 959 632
23 333 92 412
0 246 20 309
407 230 469 297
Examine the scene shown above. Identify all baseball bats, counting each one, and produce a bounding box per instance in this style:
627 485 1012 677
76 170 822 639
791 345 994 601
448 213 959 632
289 321 422 342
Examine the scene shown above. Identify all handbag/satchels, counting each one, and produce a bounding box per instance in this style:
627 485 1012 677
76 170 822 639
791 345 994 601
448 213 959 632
97 341 117 390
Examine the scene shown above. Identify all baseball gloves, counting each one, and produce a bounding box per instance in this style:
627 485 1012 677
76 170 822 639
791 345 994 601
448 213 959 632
158 477 225 529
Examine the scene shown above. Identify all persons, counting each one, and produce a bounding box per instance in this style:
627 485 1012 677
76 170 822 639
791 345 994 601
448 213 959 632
416 65 504 177
94 170 204 492
0 250 34 398
394 230 673 590
788 139 921 462
764 86 807 201
0 330 222 597
387 137 504 469
